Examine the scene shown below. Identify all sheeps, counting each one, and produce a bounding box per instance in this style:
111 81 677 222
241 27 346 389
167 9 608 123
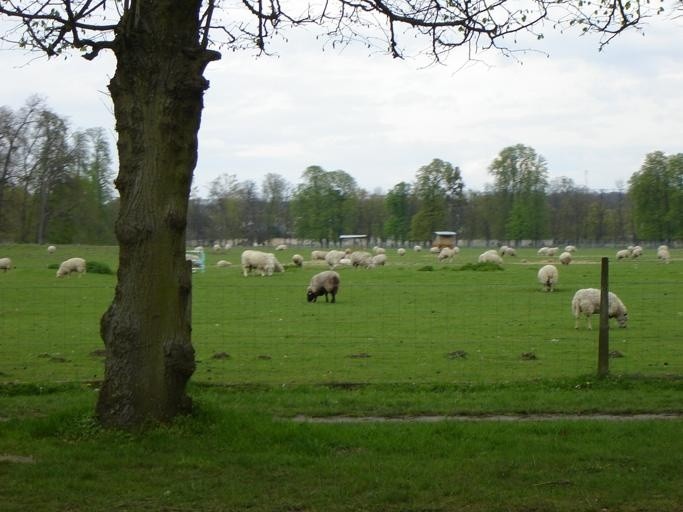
0 257 11 273
397 248 406 256
241 250 284 277
275 245 288 250
47 245 58 254
185 243 233 274
571 288 629 331
414 245 422 252
537 265 559 292
306 271 339 303
56 257 86 280
616 244 671 265
311 244 388 270
478 245 517 265
430 246 460 264
291 254 303 267
537 245 576 265
